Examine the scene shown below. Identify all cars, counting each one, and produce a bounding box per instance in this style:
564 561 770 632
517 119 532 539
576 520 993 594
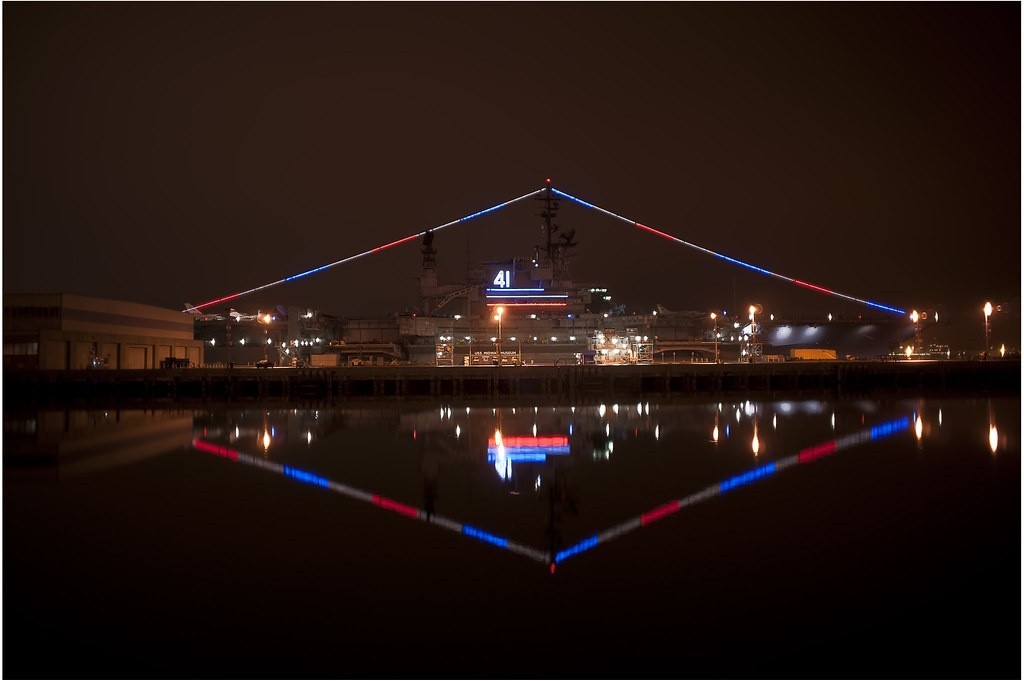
256 360 273 368
292 357 304 367
351 359 364 366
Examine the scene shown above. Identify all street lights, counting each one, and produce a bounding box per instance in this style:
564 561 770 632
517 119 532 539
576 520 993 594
983 302 992 356
710 312 718 363
750 305 756 362
497 306 503 364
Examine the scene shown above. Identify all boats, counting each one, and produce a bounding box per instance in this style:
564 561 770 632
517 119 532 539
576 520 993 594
193 306 343 365
391 178 940 363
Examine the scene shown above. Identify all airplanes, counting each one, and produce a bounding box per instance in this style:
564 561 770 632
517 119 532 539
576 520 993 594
184 302 227 320
229 308 261 322
